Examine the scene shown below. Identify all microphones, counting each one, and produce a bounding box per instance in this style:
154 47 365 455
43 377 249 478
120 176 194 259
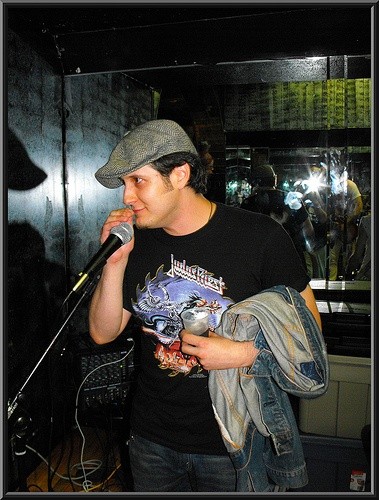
69 222 134 294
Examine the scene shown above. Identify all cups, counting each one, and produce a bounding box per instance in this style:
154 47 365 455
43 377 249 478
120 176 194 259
181 308 210 339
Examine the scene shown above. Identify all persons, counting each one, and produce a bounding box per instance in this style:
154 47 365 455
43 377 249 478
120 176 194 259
239 164 315 273
294 155 371 280
90 120 321 491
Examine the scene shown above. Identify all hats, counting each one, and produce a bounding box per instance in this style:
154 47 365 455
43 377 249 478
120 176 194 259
94 119 198 190
252 165 277 179
